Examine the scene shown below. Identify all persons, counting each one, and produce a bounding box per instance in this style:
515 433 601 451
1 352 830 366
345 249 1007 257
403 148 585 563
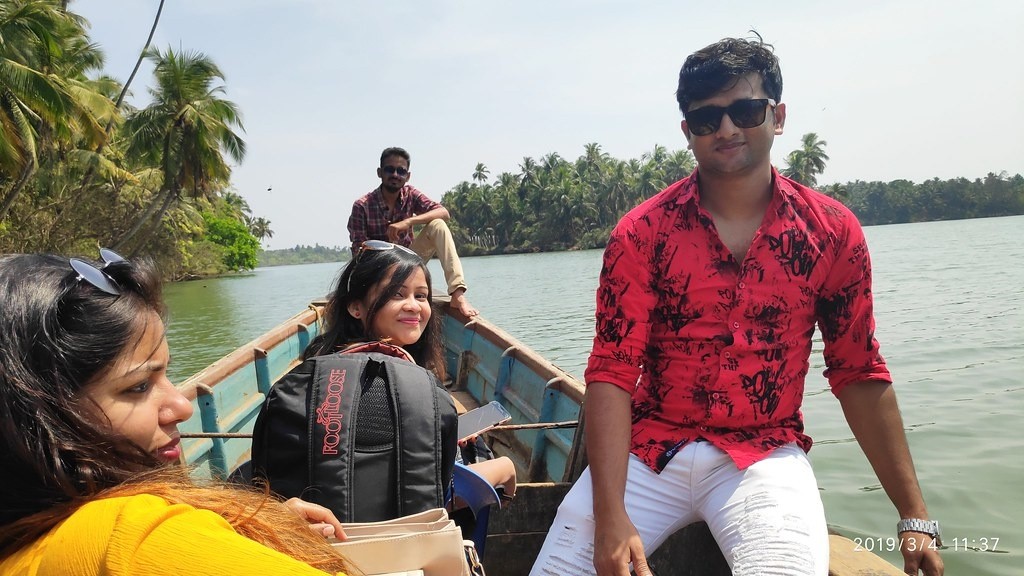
348 147 479 316
252 240 516 576
528 26 945 576
0 248 365 576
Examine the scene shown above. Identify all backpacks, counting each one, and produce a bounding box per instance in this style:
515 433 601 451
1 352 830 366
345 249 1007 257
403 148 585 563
248 340 458 524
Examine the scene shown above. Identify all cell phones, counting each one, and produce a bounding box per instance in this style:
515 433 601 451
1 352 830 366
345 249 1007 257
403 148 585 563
457 400 512 444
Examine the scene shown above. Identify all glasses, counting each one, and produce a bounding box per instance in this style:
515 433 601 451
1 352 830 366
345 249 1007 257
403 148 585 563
380 165 410 175
348 240 424 292
681 96 778 137
58 247 133 298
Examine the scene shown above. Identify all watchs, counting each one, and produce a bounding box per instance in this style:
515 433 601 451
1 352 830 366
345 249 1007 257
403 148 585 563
897 518 943 547
